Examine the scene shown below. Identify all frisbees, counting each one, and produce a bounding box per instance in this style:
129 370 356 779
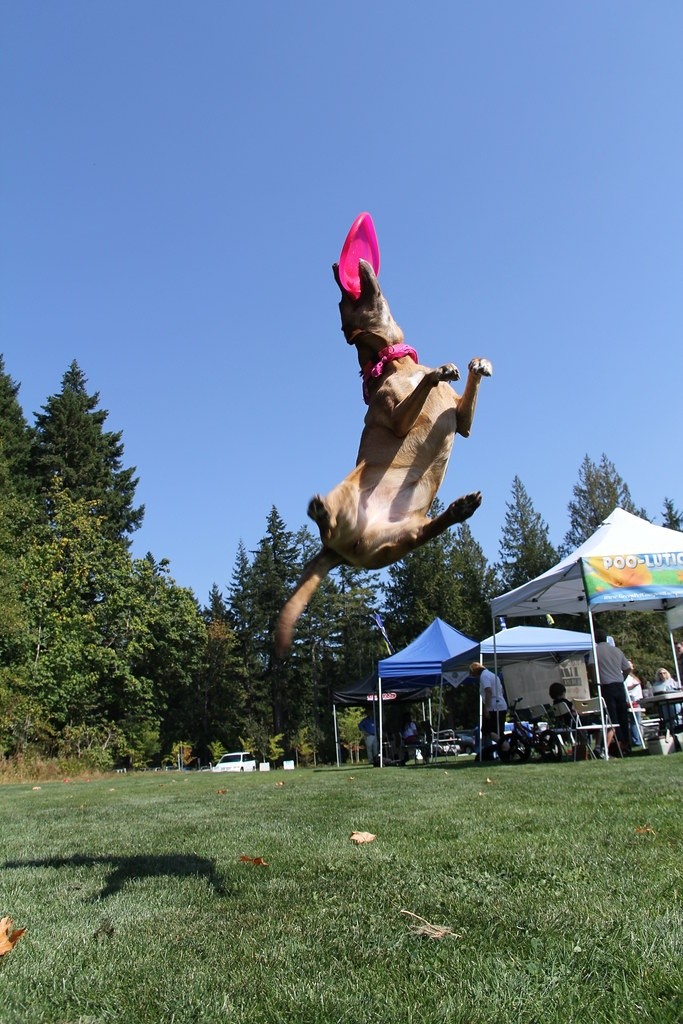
341 210 381 296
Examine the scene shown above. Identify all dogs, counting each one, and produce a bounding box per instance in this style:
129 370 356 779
271 258 495 655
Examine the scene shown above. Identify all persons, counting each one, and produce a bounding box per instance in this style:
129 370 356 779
622 659 647 747
588 630 634 758
673 641 683 688
400 711 430 766
357 711 384 765
549 683 616 761
470 661 508 762
650 667 681 738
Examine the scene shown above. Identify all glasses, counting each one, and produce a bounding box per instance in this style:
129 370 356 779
660 672 666 675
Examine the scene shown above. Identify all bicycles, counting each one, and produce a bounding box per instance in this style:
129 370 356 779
499 697 562 757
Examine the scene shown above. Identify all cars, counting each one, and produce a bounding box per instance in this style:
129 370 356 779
212 752 256 772
456 727 478 756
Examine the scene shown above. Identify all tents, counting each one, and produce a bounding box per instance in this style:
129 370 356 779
332 670 433 769
490 506 683 762
435 625 597 762
666 605 683 690
376 619 504 770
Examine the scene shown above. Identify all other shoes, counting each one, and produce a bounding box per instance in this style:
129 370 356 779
600 753 609 758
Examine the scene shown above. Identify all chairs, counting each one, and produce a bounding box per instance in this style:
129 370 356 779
572 696 624 762
394 731 429 766
547 702 597 760
429 728 458 765
528 704 574 760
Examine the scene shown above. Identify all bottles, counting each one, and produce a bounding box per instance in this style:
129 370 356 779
646 681 653 697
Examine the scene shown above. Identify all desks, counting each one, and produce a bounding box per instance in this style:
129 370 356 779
641 691 683 753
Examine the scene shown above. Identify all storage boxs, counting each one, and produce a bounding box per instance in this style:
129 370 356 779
647 732 682 755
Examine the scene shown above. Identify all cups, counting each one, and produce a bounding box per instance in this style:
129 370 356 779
643 690 648 697
